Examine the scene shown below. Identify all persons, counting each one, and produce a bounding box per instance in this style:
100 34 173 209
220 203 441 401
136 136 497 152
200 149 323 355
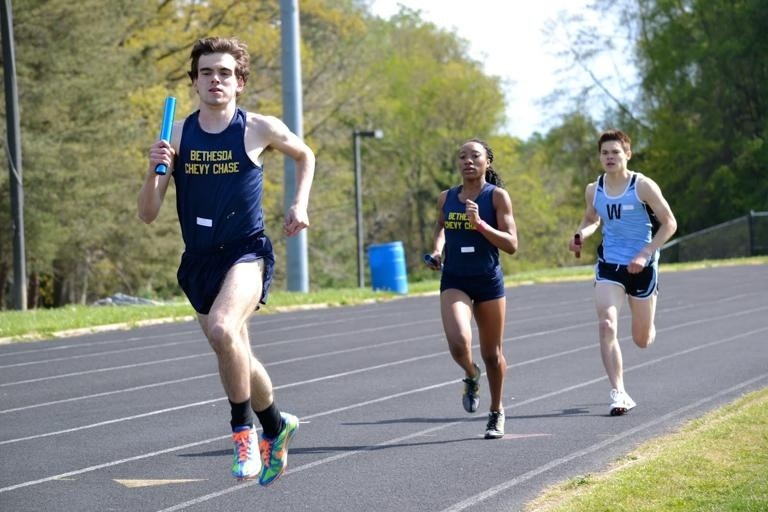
138 38 315 487
569 130 677 416
427 140 518 439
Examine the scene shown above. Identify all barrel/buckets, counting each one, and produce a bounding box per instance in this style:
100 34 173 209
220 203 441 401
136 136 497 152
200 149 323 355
364 239 407 295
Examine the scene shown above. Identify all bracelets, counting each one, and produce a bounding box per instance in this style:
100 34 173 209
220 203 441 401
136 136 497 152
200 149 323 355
476 221 486 232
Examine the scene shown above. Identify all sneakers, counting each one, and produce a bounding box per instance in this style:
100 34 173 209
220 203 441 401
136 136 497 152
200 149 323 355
463 363 481 414
484 405 506 438
609 393 637 415
232 427 260 479
260 411 301 489
